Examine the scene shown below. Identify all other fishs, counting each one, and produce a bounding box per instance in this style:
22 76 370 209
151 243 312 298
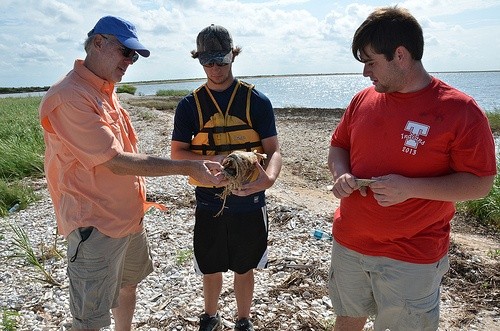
220 150 267 199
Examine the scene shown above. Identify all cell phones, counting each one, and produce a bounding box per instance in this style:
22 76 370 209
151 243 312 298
79 225 93 241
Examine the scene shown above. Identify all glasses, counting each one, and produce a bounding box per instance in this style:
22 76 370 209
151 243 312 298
97 32 139 63
198 52 233 69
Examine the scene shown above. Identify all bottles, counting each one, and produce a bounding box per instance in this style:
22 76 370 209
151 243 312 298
309 229 333 240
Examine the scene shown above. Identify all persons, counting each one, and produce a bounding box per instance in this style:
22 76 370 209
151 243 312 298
38 16 225 331
169 23 282 331
328 6 497 331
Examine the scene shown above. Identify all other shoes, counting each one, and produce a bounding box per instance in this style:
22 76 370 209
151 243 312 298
234 318 254 331
197 312 222 331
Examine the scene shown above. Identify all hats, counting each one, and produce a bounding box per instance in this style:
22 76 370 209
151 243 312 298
88 15 150 58
196 23 232 53
